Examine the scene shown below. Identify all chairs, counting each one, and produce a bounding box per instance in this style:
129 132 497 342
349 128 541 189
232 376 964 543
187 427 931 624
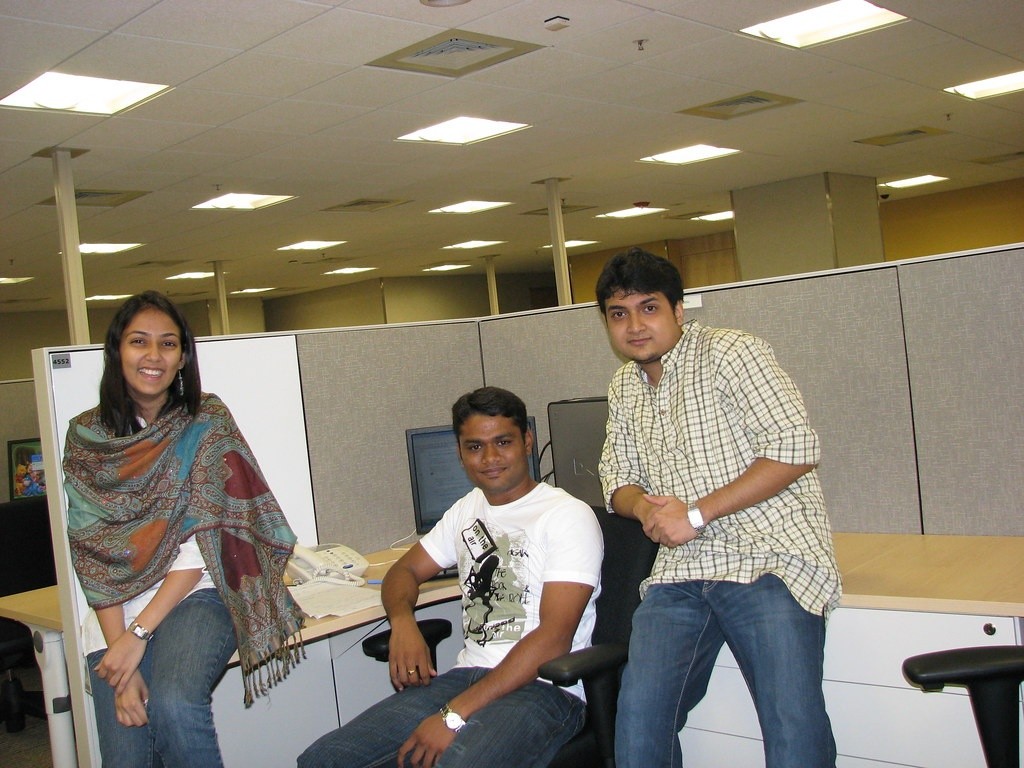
361 505 661 768
903 645 1024 768
0 494 57 732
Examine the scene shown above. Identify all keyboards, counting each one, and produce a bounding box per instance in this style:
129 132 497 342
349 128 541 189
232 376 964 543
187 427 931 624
425 563 459 582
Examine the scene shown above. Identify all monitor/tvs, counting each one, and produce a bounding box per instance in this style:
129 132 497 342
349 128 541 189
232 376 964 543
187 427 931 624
405 416 540 535
7 438 48 501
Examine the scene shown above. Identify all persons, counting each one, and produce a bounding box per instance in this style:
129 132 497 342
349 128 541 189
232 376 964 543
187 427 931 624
297 385 607 768
62 289 296 768
594 247 843 768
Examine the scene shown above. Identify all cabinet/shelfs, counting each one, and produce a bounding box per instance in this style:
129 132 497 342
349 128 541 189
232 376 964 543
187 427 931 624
678 532 1024 768
212 594 465 768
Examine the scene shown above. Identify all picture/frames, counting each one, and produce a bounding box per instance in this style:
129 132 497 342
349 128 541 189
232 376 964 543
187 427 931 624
7 437 46 501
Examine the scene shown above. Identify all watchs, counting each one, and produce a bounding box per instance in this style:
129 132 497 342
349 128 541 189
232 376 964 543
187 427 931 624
438 702 466 734
129 622 154 642
686 501 707 538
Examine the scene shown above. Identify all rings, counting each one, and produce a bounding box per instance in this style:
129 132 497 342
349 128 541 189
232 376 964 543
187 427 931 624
407 670 415 675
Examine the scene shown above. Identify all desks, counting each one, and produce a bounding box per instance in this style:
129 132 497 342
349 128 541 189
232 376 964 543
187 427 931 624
0 584 78 768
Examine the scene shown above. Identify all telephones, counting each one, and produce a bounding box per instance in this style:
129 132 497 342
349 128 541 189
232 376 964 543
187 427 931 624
285 542 371 587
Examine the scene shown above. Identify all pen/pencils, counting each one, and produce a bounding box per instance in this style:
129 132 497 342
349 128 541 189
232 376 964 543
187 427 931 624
367 579 382 583
